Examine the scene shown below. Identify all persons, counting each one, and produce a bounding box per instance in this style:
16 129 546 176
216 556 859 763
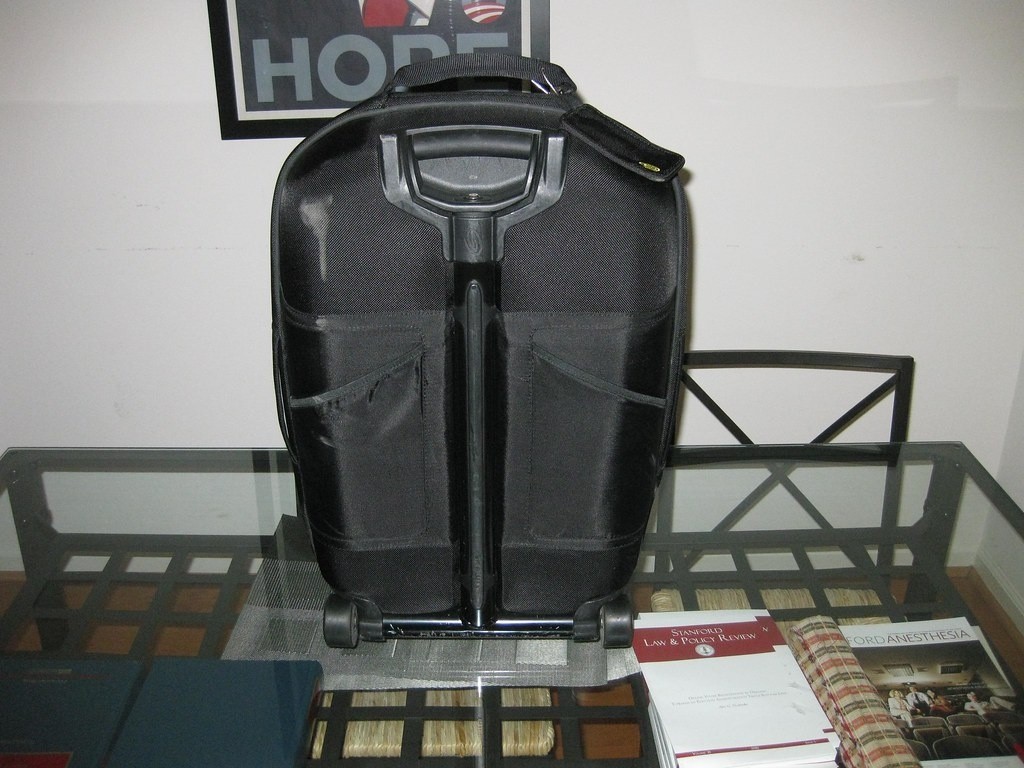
887 683 994 733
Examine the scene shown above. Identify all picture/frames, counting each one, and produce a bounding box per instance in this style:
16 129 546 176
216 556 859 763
207 0 550 139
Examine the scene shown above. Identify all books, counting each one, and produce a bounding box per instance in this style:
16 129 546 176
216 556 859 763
1 647 323 767
827 614 1023 768
632 609 841 768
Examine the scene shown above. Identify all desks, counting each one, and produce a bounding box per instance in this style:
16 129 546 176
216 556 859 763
0 435 1023 768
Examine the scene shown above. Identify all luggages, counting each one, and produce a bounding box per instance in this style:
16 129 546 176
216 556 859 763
270 52 695 651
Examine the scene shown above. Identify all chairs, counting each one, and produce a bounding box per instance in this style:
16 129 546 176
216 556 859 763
656 346 914 633
314 685 556 764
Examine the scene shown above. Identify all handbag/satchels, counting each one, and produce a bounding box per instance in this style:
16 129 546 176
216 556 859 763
923 700 929 714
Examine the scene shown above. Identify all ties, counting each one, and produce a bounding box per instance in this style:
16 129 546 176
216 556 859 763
913 693 923 707
